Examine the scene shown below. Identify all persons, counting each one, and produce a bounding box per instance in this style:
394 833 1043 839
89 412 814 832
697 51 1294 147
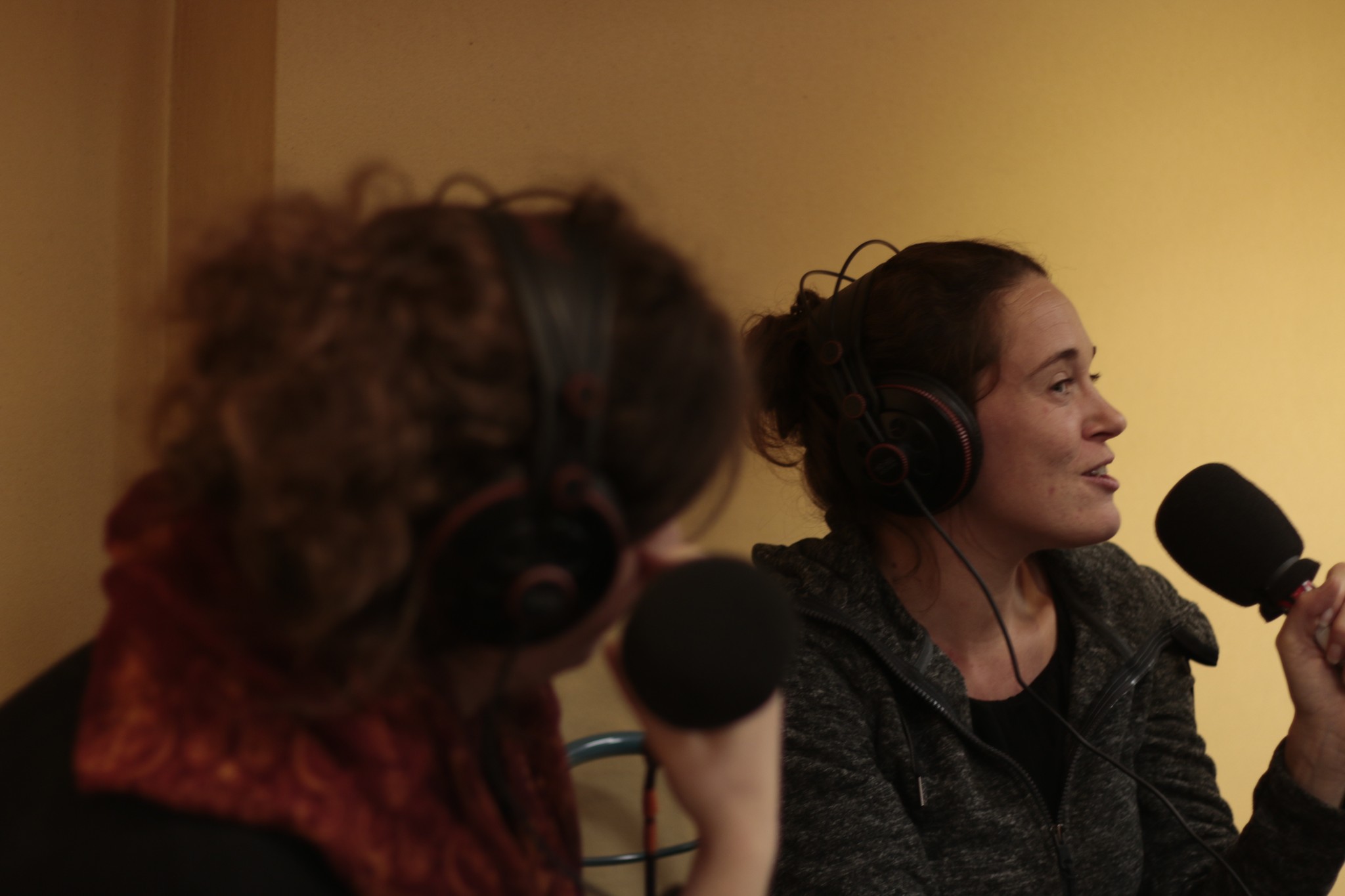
745 240 1344 896
1 156 784 896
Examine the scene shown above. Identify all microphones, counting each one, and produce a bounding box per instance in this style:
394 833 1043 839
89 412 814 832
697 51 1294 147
627 558 792 733
1152 462 1332 650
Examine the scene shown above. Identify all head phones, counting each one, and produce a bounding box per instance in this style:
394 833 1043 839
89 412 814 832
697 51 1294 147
795 237 977 517
431 168 626 649
